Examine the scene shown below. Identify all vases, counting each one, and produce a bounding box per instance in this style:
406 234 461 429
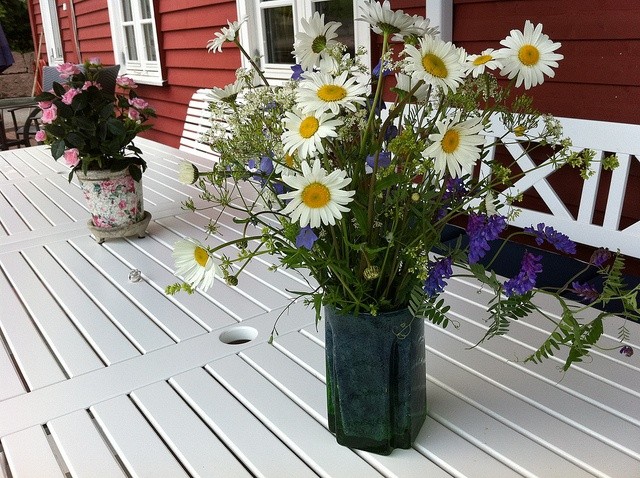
323 296 426 454
73 161 151 243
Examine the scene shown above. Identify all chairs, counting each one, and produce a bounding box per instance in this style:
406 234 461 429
8 62 85 148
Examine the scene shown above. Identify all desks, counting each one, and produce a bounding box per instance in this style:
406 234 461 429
1 136 640 478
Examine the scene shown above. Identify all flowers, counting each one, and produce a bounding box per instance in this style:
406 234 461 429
165 0 640 324
35 57 159 182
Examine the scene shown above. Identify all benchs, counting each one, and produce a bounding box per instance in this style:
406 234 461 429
177 89 282 171
375 103 640 324
